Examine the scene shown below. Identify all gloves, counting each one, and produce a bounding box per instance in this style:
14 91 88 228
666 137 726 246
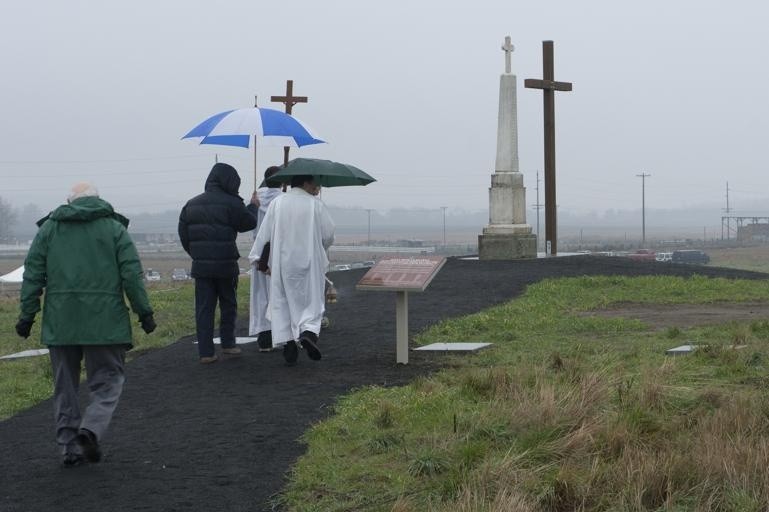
136 311 158 335
14 318 36 342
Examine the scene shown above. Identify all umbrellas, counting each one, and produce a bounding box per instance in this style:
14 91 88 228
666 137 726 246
180 94 327 191
257 156 380 199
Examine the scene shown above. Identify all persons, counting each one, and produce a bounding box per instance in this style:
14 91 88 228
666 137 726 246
248 175 337 365
15 181 157 463
248 165 286 353
178 162 261 364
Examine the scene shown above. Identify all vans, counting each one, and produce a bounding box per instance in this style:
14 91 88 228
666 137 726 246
576 248 711 265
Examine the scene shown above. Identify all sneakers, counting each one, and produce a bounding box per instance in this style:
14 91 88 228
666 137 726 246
221 345 242 355
197 352 218 365
255 344 273 354
76 425 105 465
299 330 323 362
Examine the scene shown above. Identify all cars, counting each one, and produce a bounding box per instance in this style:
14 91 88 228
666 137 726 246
334 261 376 270
239 268 249 276
146 271 162 281
172 268 188 281
